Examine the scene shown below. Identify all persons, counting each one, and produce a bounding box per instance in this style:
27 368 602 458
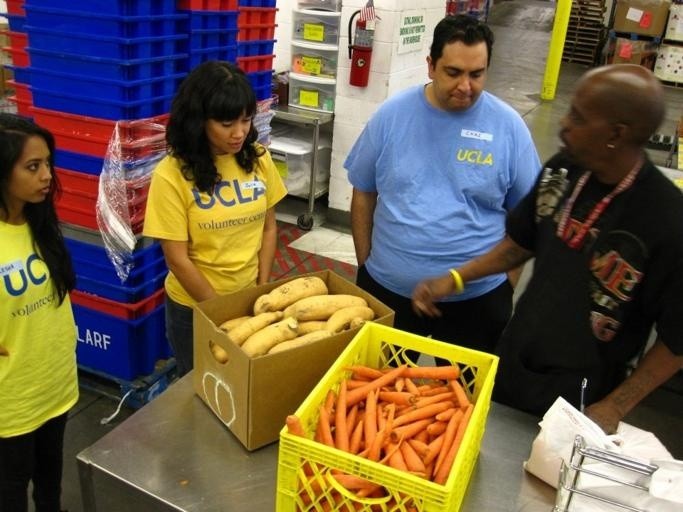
141 62 288 378
411 64 683 436
0 110 81 512
344 12 543 392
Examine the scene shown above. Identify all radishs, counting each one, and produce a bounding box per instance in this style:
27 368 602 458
212 276 375 365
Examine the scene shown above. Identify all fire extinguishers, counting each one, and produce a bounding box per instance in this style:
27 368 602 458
349 5 375 88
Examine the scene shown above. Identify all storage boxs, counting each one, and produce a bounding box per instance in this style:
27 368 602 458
288 0 342 115
189 267 395 454
267 131 331 194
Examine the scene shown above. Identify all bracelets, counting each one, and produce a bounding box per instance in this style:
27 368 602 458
446 265 466 297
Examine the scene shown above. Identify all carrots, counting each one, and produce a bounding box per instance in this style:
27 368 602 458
286 364 474 512
